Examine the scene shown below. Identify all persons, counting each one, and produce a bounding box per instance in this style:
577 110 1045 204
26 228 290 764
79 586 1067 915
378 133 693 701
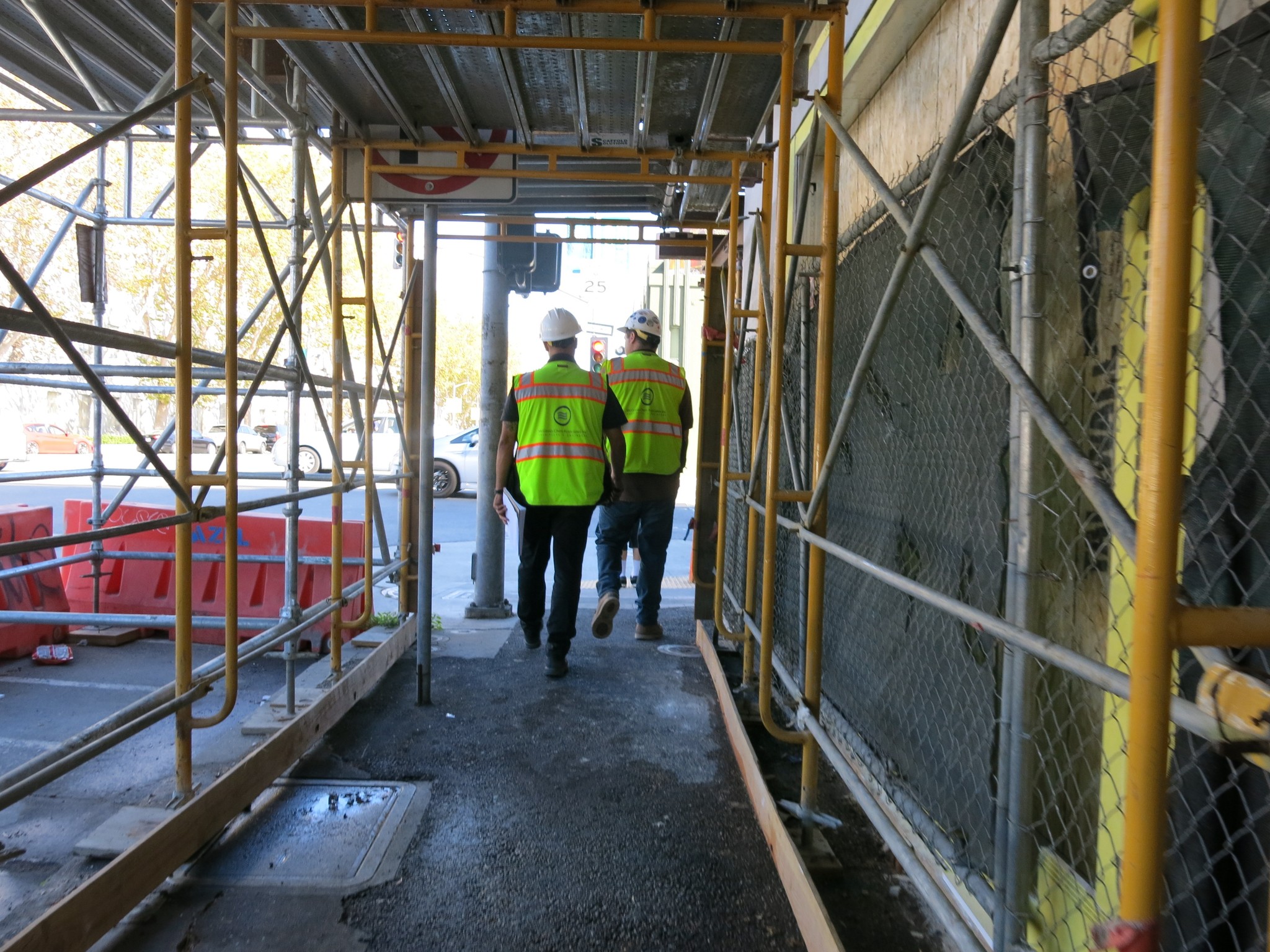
591 308 694 640
493 307 628 676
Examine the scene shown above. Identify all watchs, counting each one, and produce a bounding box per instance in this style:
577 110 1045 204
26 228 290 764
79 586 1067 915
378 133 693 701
493 489 503 494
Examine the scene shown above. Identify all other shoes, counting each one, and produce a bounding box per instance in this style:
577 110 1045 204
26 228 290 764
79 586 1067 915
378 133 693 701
630 575 638 587
520 618 541 649
620 575 627 588
634 621 664 640
592 593 620 639
544 656 568 677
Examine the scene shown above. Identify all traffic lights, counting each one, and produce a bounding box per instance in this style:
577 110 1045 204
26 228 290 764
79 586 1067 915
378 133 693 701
590 337 608 373
393 232 403 269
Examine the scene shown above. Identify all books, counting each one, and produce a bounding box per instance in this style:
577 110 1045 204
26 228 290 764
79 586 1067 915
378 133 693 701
501 487 526 556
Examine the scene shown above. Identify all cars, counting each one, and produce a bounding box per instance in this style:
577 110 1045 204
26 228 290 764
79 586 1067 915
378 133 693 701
389 424 518 498
23 423 93 454
272 413 402 474
136 428 217 455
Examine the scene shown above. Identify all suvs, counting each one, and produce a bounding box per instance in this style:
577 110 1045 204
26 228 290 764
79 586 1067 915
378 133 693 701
206 424 267 454
254 425 285 452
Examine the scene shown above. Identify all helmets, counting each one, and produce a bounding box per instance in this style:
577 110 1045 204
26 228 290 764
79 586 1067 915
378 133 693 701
617 309 662 338
541 307 582 342
